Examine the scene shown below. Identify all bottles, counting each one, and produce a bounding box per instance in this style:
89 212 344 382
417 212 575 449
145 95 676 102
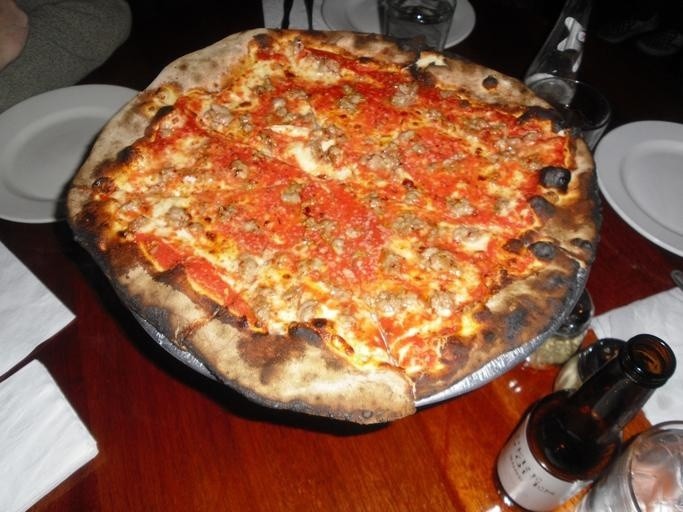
522 3 594 110
489 284 676 507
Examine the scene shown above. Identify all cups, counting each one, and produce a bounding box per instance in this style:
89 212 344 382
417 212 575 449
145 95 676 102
520 78 618 146
574 419 682 512
381 0 458 53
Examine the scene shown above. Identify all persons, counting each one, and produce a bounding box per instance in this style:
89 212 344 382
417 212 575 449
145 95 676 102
0 0 132 112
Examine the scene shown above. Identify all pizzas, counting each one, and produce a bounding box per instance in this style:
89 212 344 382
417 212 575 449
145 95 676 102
63 26 606 426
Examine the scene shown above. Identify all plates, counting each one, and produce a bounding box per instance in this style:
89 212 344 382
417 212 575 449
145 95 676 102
0 80 147 226
93 237 591 419
320 0 477 49
596 115 683 259
